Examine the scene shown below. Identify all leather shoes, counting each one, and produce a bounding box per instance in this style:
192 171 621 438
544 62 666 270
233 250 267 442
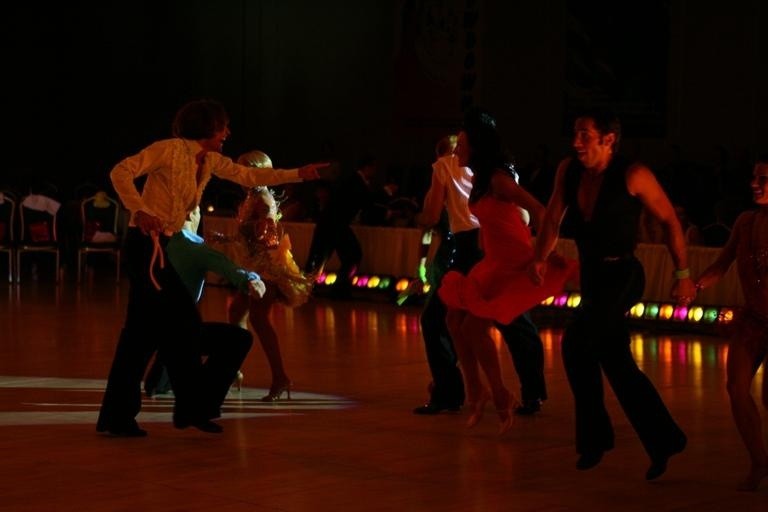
174 418 224 433
646 443 686 480
515 399 540 414
414 401 463 413
576 445 614 470
110 425 147 437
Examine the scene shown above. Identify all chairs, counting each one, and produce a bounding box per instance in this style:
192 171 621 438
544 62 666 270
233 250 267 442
77 195 122 286
16 196 59 283
0 195 14 282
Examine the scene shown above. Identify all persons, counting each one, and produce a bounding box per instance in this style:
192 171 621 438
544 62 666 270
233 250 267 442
301 160 399 272
444 107 552 438
410 138 485 415
208 150 309 401
678 156 767 492
522 108 697 482
95 96 332 439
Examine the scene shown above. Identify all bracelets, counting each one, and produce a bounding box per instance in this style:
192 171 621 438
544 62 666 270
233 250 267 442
671 268 691 281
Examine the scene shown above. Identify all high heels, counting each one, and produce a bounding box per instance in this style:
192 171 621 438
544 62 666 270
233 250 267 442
262 381 291 401
233 370 243 391
469 389 522 434
